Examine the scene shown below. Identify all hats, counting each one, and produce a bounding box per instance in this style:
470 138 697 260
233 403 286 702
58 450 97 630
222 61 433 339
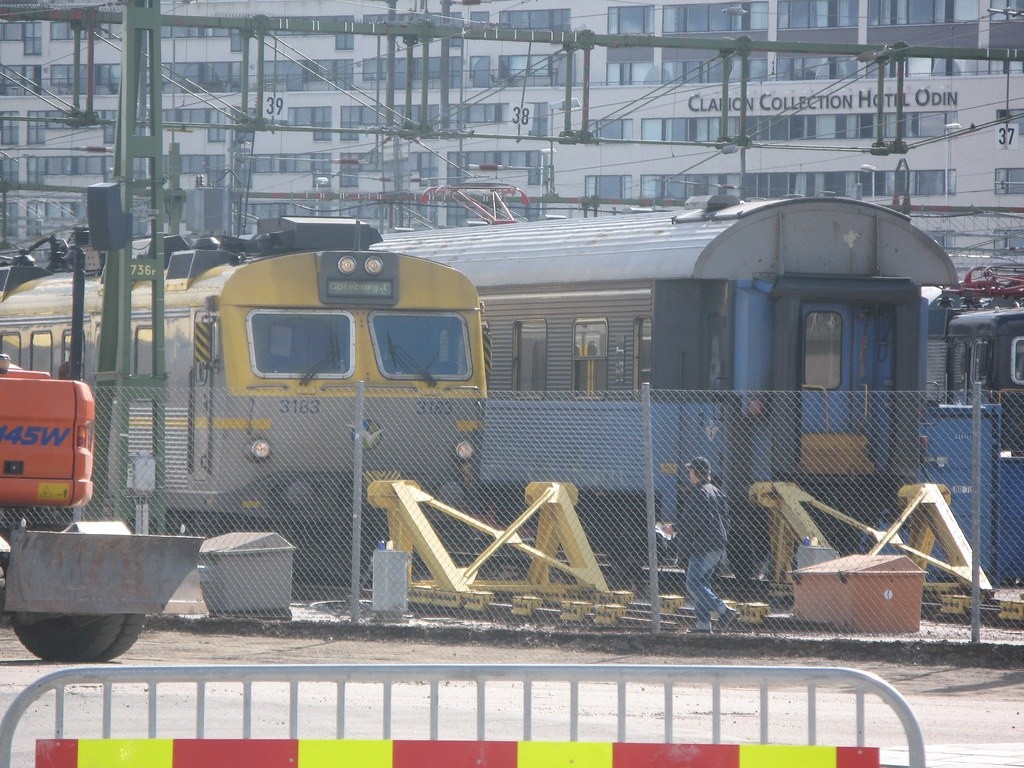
685 458 712 474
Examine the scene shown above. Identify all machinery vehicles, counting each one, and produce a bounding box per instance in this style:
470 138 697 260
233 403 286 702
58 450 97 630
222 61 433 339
1 354 206 666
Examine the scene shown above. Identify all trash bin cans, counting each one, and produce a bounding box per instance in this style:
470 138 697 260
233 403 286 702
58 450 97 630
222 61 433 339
369 540 409 623
797 537 836 568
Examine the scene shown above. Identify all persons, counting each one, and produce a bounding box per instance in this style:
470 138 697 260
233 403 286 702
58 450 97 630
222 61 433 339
661 458 740 632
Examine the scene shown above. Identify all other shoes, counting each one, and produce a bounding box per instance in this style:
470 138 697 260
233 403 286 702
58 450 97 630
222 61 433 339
686 626 711 633
714 609 740 633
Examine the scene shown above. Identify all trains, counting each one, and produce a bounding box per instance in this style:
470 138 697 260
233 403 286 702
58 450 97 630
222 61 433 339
354 193 1023 602
1 200 490 588
